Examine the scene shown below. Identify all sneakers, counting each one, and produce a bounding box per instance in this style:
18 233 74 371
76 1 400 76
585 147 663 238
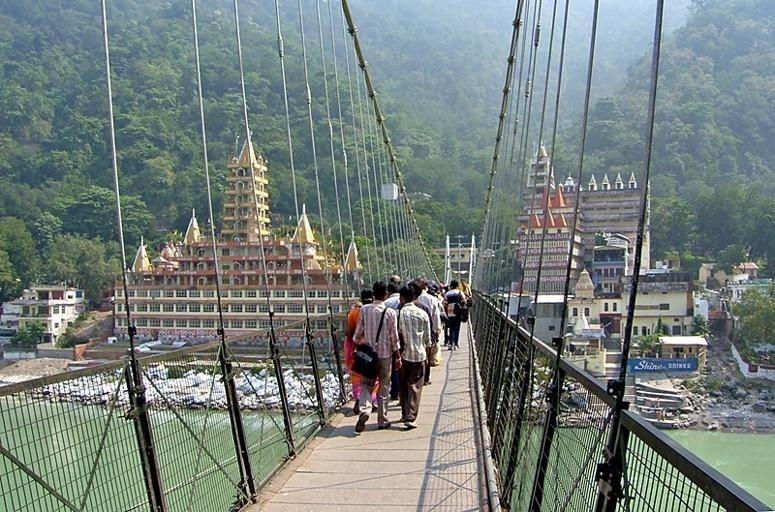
443 342 459 350
379 422 391 430
353 399 359 415
423 380 432 386
401 417 419 428
355 412 369 433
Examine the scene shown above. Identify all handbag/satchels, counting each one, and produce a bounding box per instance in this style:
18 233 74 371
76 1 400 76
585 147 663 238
351 343 378 379
429 342 442 366
454 303 462 315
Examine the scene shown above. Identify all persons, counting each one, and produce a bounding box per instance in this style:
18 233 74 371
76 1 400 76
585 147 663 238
341 272 474 434
